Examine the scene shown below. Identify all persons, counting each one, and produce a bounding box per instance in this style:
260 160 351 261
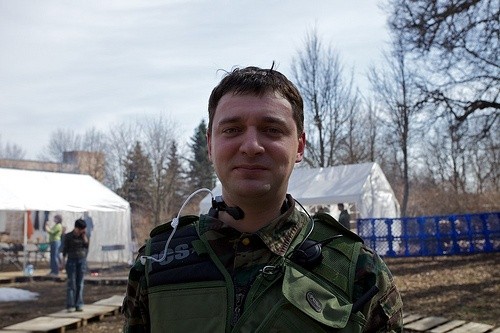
45 214 63 278
337 203 350 229
120 60 405 333
60 217 89 312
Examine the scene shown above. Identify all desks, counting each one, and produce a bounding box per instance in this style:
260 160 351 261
0 250 50 270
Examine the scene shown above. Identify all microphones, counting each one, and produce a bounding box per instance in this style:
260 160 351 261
215 196 245 221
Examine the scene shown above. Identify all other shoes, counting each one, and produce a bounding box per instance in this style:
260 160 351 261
76 307 83 311
68 307 76 311
47 271 59 277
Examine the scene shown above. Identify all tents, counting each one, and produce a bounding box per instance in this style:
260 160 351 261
197 160 402 257
0 168 132 264
